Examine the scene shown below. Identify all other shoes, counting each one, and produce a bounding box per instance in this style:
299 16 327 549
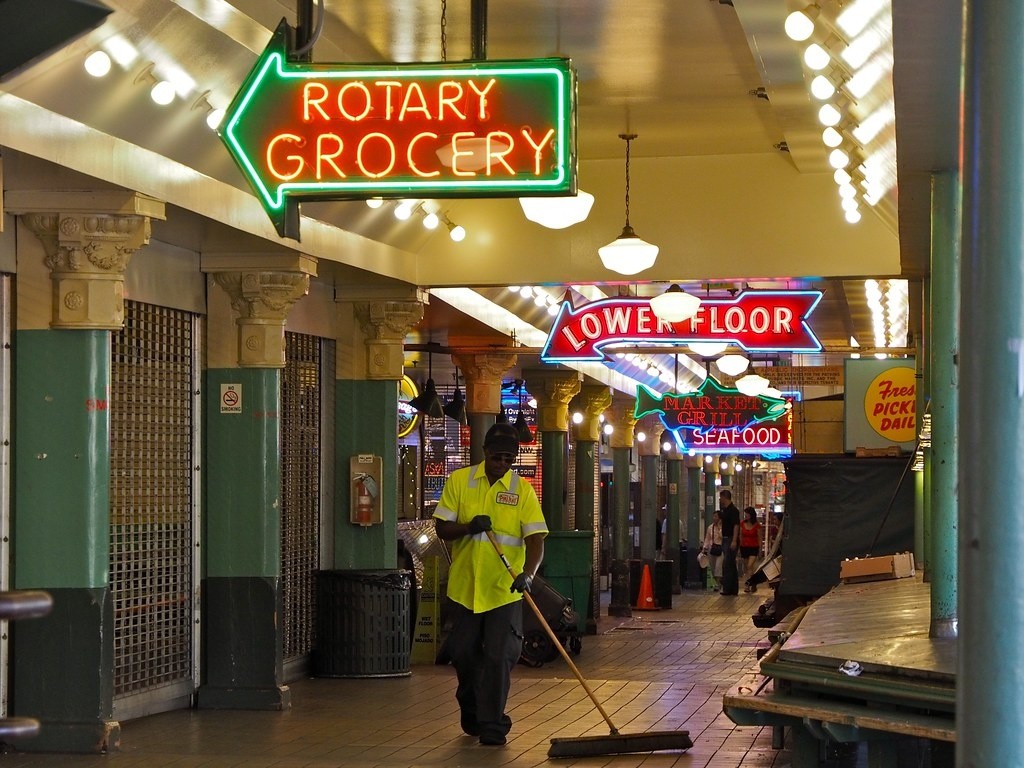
461 703 479 736
480 736 506 744
715 583 721 591
744 586 757 593
720 591 738 596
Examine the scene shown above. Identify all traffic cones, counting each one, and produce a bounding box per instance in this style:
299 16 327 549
633 565 662 611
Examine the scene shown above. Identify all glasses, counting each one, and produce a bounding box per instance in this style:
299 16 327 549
487 449 516 463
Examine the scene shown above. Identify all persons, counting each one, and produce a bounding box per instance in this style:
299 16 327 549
702 510 724 591
771 513 783 557
719 489 740 595
660 503 685 595
430 423 550 745
740 506 763 593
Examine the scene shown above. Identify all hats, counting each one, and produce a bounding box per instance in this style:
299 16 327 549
485 423 519 456
662 504 667 510
697 552 707 568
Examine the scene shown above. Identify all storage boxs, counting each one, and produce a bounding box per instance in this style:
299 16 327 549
840 554 914 585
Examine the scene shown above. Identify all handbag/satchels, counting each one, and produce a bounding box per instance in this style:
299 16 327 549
753 557 764 573
711 544 722 556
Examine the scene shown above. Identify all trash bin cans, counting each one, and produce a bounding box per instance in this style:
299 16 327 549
630 559 674 609
311 567 413 679
540 530 595 656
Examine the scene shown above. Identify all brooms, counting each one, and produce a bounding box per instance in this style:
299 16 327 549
485 525 695 760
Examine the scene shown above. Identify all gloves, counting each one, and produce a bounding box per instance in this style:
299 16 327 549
510 573 532 593
469 515 492 535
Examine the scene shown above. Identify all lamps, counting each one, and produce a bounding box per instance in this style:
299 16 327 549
518 190 595 228
408 342 444 418
735 354 770 396
759 352 781 398
443 365 468 425
598 134 660 275
497 378 533 443
650 283 702 322
715 344 750 376
686 283 728 356
